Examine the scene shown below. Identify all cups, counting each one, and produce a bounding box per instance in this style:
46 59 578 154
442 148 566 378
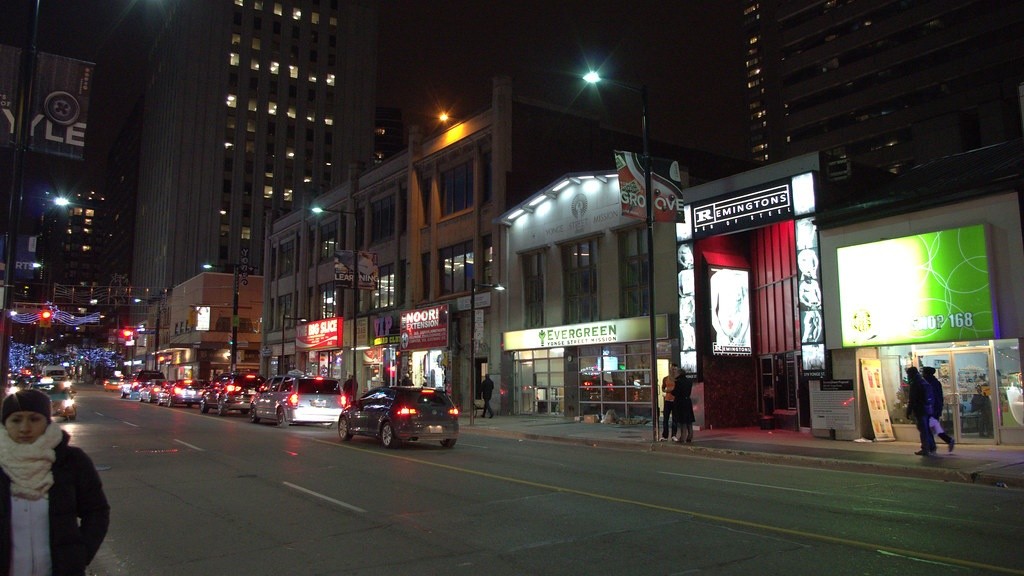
874 423 880 434
882 399 886 410
886 422 891 434
876 400 881 410
880 422 885 433
871 400 876 410
874 373 881 388
867 373 874 388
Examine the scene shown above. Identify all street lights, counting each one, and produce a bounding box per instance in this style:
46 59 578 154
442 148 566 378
282 315 308 375
133 298 162 371
582 69 662 442
203 262 240 371
469 277 508 426
310 207 359 402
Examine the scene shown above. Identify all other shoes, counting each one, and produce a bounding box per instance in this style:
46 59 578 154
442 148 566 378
671 436 679 442
913 448 937 456
948 439 955 453
488 412 495 418
659 437 668 442
678 437 692 443
481 414 485 418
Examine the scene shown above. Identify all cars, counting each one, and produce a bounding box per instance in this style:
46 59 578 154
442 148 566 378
103 369 210 409
4 364 76 423
336 385 459 448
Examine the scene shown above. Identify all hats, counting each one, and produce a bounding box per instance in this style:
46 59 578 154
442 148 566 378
1 389 53 425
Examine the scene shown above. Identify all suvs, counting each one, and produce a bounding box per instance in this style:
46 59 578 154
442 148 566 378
199 371 268 417
249 375 347 429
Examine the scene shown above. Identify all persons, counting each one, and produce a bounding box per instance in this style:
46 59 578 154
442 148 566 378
480 374 494 418
906 367 954 456
0 387 111 576
796 217 825 369
709 268 751 348
658 363 696 443
677 242 697 374
402 374 414 386
343 374 358 402
